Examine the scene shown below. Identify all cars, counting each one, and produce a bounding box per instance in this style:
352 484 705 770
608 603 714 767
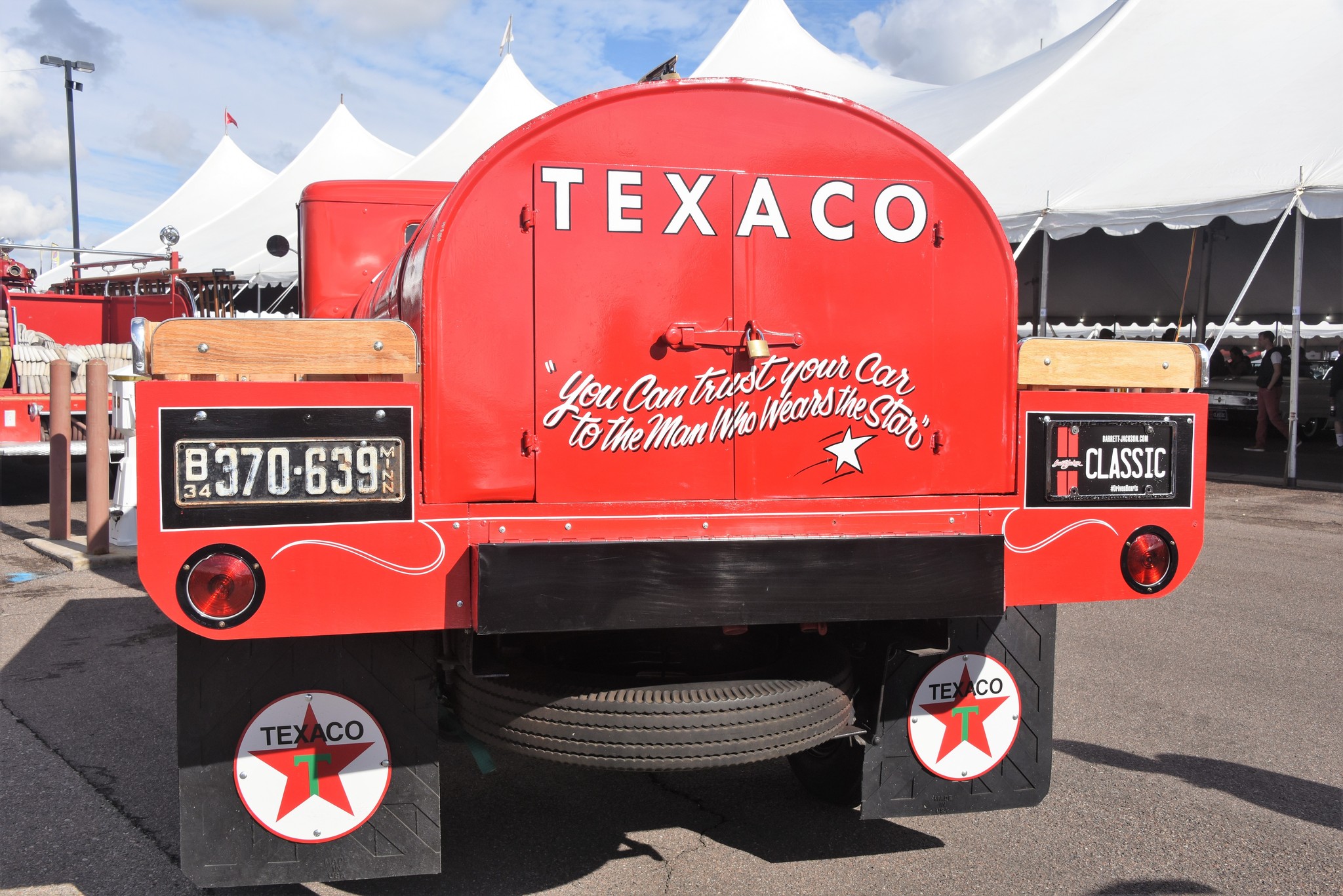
1209 376 1319 422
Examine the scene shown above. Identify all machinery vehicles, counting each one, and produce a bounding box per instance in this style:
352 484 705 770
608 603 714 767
135 1 1203 887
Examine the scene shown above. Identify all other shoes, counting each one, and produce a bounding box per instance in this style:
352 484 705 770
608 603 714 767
1331 444 1343 451
1284 440 1302 453
1244 444 1265 452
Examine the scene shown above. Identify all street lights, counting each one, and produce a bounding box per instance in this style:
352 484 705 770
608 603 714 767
40 55 94 294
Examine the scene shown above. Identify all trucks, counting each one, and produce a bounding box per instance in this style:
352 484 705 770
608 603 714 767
0 227 233 499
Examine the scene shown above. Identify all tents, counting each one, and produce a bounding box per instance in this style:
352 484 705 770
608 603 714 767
7 134 278 295
110 94 416 272
1017 321 1343 347
225 54 559 323
689 0 949 104
864 0 1343 482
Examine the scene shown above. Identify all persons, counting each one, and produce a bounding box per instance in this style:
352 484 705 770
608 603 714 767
1281 345 1292 377
1161 328 1177 342
1099 328 1116 339
1224 347 1255 376
1327 340 1343 450
1244 331 1302 452
1205 338 1225 379
1299 346 1311 378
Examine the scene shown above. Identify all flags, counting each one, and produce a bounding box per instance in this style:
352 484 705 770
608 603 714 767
500 15 514 56
225 108 239 128
